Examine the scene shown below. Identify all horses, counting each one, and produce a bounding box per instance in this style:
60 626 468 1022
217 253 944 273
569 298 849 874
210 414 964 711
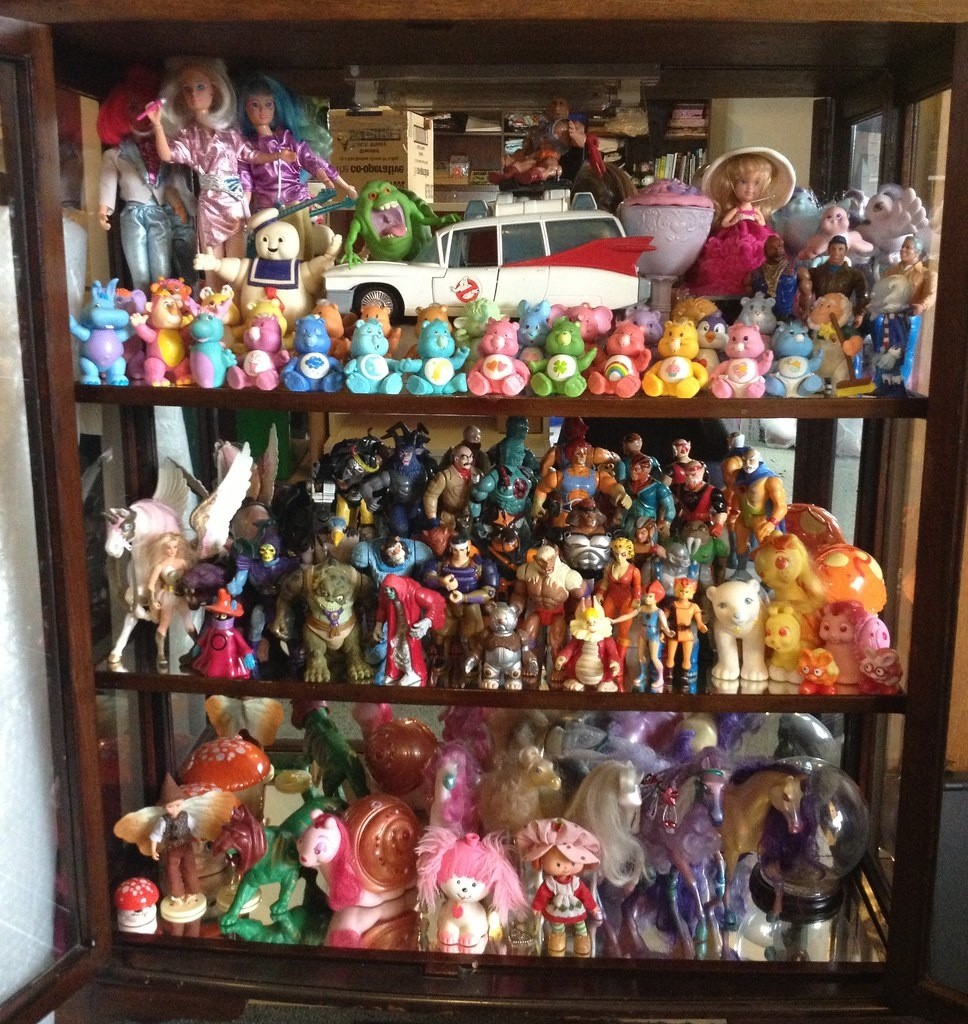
101 498 193 670
598 745 804 962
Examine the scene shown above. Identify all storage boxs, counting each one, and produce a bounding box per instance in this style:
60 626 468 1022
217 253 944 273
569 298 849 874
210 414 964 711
326 107 435 207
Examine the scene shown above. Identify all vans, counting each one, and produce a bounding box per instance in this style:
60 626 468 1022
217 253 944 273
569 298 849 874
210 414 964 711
321 213 641 323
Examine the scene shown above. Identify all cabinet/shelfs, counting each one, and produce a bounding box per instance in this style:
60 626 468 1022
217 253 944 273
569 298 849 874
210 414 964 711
0 0 966 1024
